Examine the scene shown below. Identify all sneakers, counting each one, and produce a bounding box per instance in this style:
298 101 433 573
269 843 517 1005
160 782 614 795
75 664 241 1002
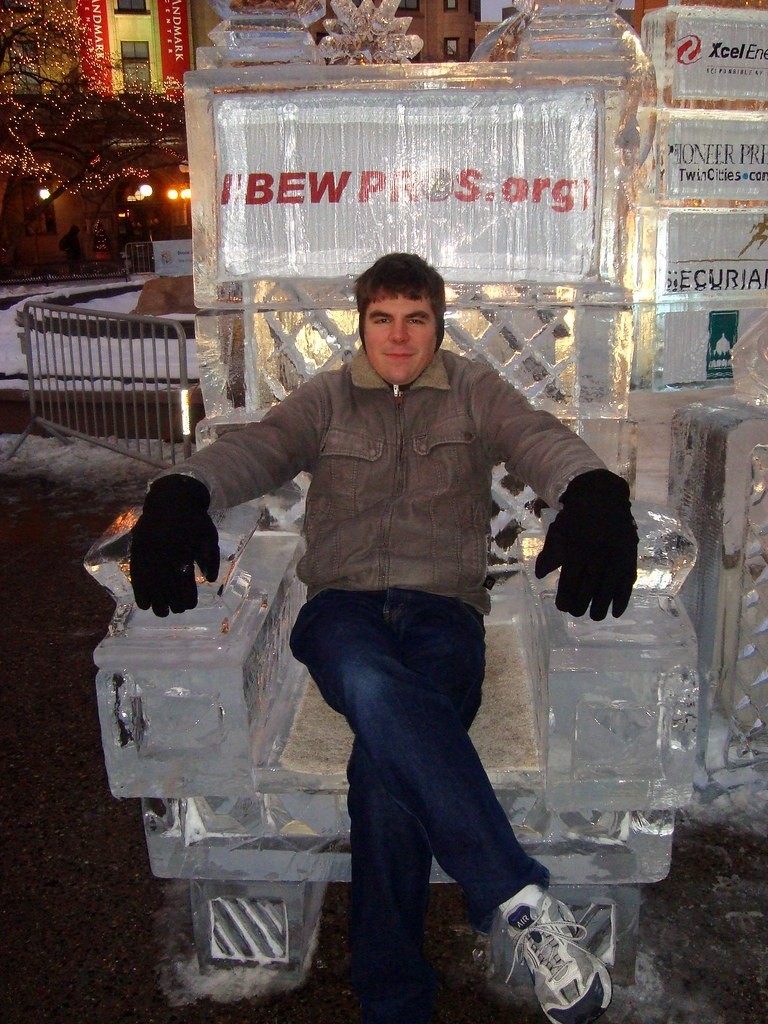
500 886 613 1024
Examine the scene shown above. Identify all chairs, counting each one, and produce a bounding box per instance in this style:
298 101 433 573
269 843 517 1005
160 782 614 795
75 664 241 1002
79 59 699 989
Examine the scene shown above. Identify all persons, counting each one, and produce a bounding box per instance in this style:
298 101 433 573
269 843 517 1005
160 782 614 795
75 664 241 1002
131 252 641 1023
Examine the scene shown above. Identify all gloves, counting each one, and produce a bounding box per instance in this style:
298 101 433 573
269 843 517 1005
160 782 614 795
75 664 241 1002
129 473 219 617
535 468 638 622
